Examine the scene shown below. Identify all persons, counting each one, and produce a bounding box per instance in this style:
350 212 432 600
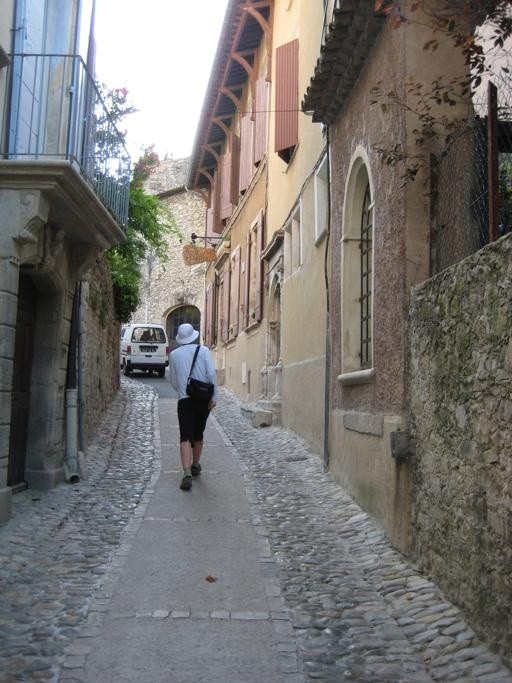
167 322 220 489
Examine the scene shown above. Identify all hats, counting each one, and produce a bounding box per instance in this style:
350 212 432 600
175 321 201 345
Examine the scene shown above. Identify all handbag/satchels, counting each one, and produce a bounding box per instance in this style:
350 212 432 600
185 376 215 402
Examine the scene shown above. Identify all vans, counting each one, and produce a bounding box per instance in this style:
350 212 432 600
120 324 170 376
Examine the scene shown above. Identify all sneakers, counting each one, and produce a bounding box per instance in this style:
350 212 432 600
179 475 194 490
191 461 202 476
